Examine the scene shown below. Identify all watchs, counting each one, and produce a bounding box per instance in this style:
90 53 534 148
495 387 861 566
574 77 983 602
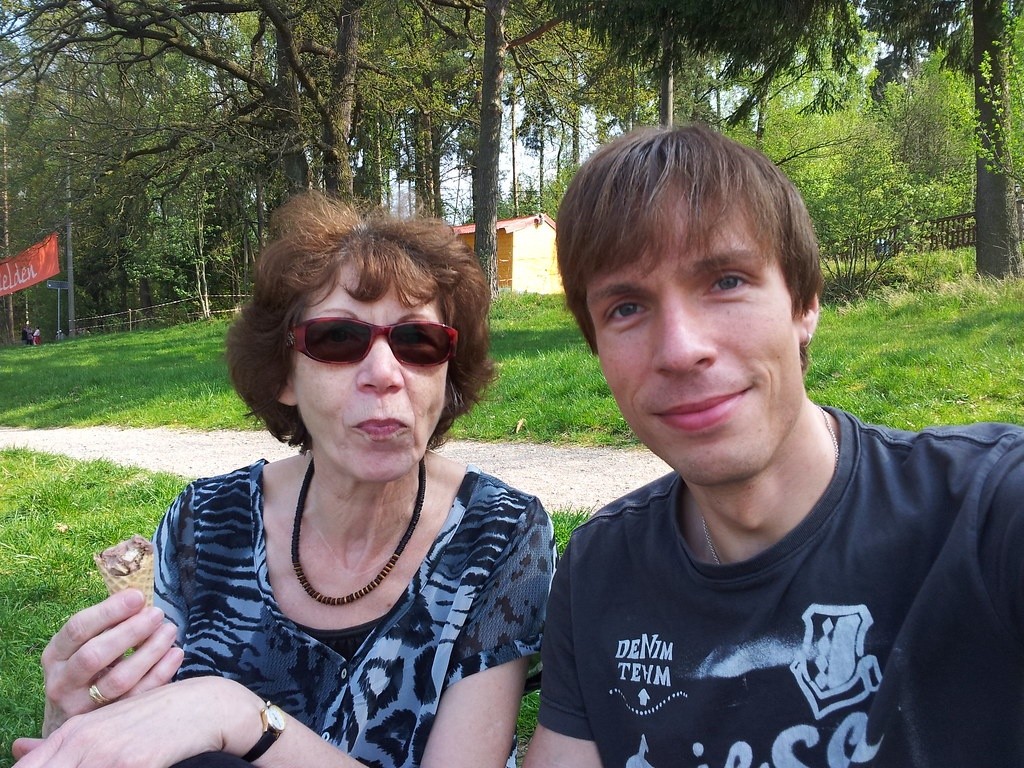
241 699 286 762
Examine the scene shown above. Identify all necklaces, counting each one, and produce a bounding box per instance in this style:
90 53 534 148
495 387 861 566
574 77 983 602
290 456 426 606
702 406 839 566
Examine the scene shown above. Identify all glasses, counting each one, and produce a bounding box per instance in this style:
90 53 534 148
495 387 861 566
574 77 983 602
284 317 459 367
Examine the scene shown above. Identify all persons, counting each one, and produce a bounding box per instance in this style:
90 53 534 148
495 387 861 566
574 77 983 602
10 201 559 768
55 330 64 340
522 124 1024 768
23 321 32 345
33 325 40 345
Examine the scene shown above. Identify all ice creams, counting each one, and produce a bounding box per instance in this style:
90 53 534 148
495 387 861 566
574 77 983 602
93 534 156 608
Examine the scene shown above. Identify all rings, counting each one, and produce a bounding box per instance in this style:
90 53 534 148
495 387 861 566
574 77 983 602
88 675 114 707
